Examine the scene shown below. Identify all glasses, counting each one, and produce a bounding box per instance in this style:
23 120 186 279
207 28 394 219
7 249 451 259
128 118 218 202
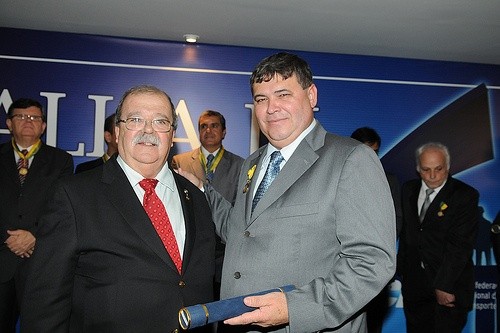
9 114 44 122
115 117 175 132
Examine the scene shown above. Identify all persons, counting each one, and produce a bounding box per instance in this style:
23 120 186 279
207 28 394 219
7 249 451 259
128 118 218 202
172 109 244 205
75 112 118 174
0 100 73 322
171 53 398 333
349 127 382 333
18 86 217 333
401 141 480 333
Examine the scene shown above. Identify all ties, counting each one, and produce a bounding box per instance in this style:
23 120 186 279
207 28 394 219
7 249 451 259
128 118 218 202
420 189 435 223
204 153 215 193
138 178 183 274
18 147 29 184
251 150 284 214
